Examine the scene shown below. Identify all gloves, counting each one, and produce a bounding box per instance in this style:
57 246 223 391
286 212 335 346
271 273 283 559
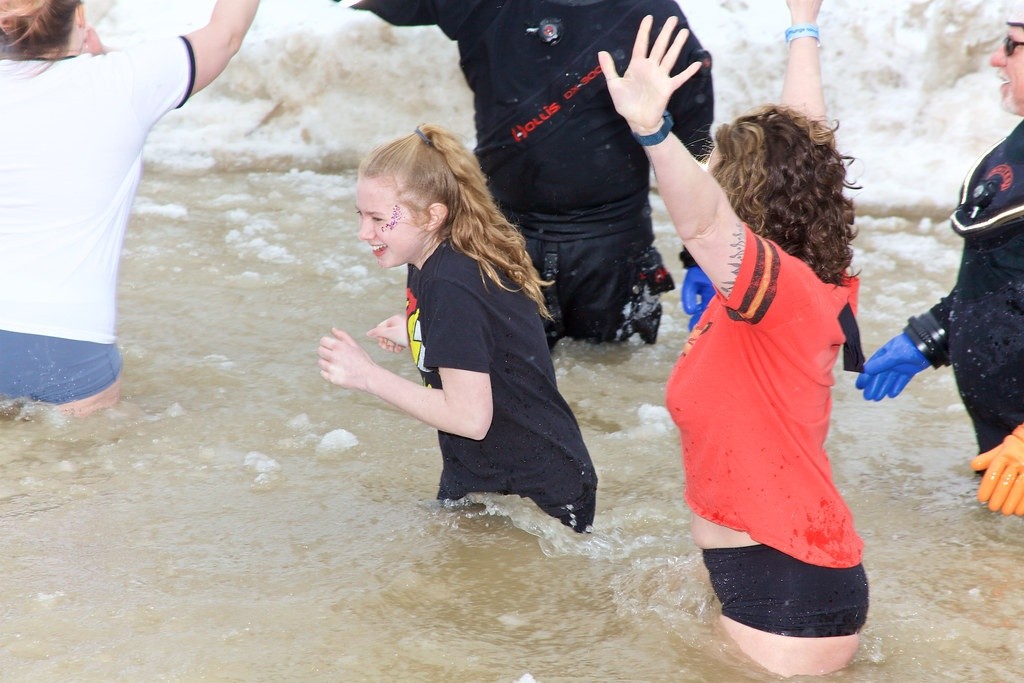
853 333 929 401
975 423 1024 517
683 265 714 333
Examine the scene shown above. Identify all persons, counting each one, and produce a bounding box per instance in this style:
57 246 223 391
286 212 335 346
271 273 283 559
324 0 716 349
315 124 602 541
593 2 876 677
0 0 270 419
850 5 1024 524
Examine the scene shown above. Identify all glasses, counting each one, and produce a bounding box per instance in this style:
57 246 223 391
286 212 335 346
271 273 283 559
1005 36 1024 55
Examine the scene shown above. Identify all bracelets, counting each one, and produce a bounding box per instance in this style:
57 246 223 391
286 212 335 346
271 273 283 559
633 108 676 146
784 22 822 49
